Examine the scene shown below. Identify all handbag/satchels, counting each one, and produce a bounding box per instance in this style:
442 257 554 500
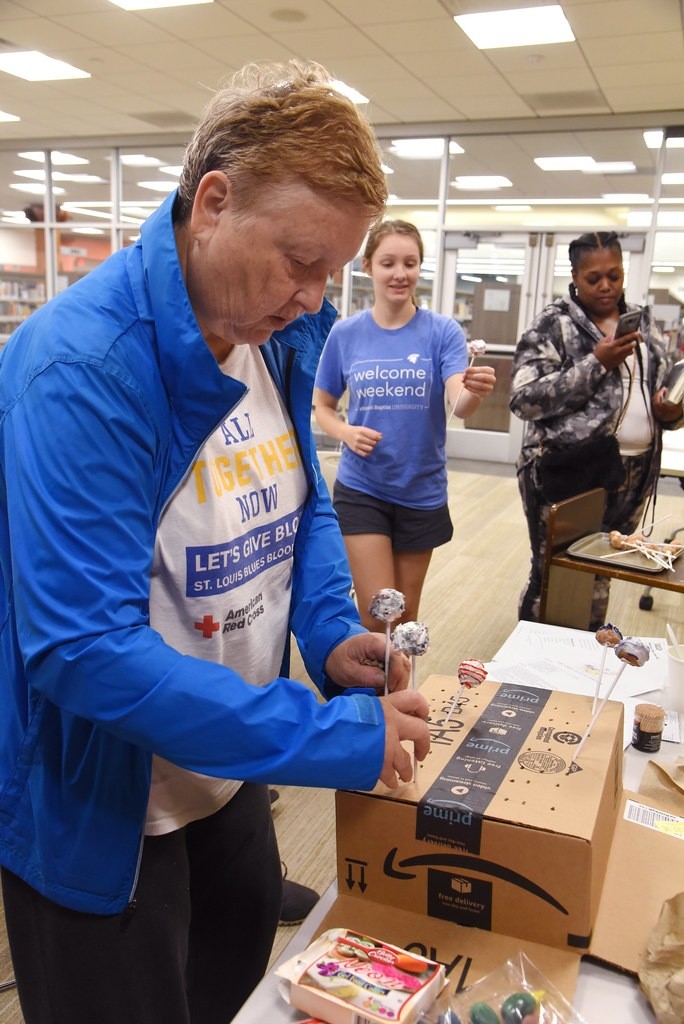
541 434 627 505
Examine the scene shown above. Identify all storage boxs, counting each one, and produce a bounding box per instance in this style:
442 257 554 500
306 673 684 1024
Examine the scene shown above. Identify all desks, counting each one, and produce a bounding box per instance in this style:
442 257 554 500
550 511 684 595
230 618 684 1024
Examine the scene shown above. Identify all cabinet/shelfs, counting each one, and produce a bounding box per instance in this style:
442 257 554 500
0 271 48 340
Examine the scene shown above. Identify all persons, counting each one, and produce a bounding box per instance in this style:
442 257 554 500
269 788 319 927
0 57 430 1024
315 217 498 658
509 230 684 633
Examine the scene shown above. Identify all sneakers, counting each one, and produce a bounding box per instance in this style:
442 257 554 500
280 864 321 928
267 790 281 814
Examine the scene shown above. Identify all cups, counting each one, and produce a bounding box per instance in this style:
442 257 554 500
632 713 664 753
667 645 684 713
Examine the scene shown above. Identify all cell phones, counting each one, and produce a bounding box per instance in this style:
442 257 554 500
612 310 643 342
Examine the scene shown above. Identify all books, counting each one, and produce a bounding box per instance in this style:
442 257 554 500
0 279 47 334
660 360 684 407
